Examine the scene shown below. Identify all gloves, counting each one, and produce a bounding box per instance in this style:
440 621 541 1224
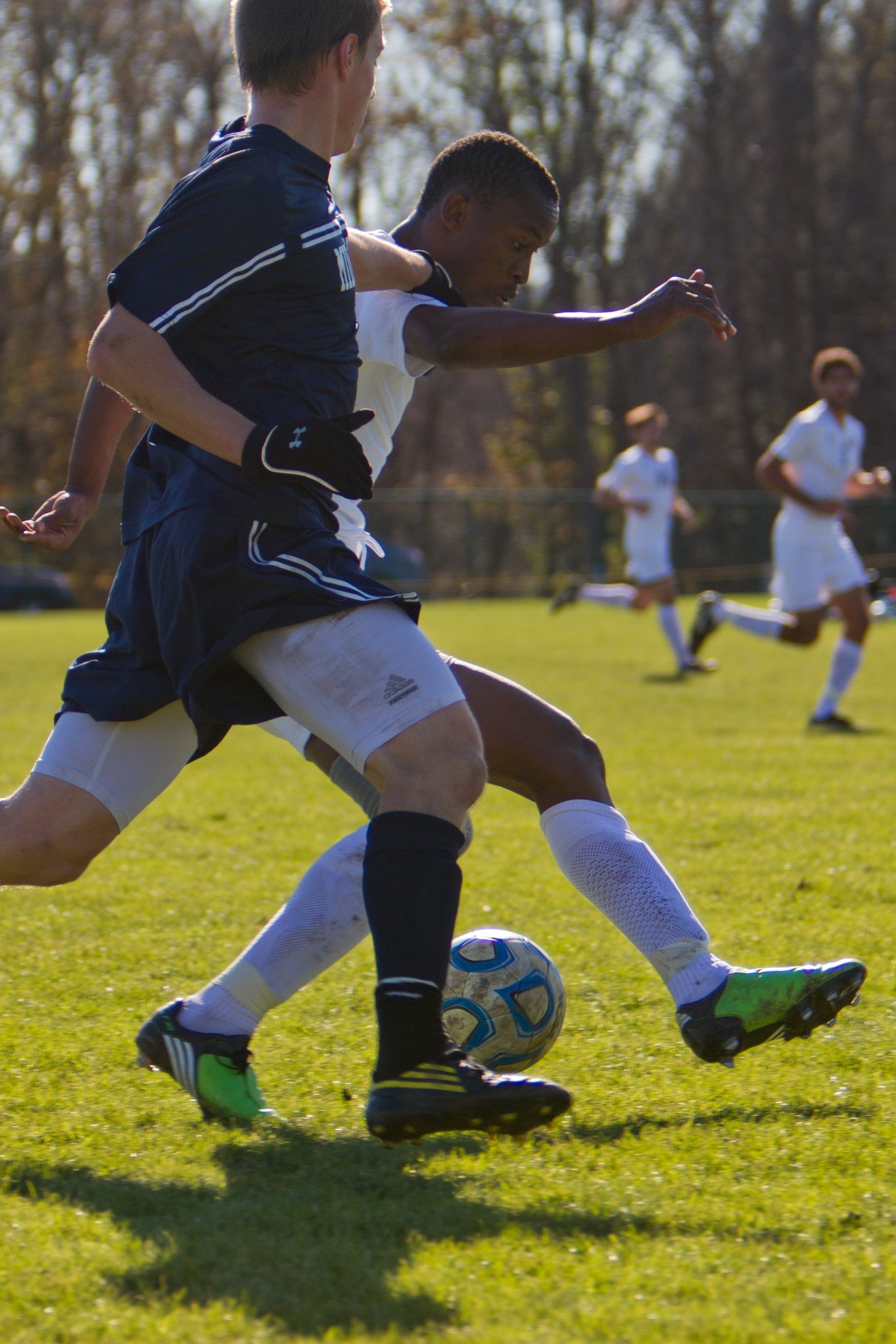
243 408 375 501
402 249 467 308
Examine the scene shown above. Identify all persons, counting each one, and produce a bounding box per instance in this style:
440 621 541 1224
0 0 575 1146
552 399 718 676
687 347 895 736
0 130 868 1136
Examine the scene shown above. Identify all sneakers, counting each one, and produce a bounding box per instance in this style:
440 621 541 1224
676 957 868 1067
365 1025 572 1143
549 573 583 610
807 713 851 731
135 997 267 1132
692 590 717 652
678 659 719 679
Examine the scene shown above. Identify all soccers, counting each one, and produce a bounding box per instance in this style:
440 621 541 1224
440 928 569 1076
